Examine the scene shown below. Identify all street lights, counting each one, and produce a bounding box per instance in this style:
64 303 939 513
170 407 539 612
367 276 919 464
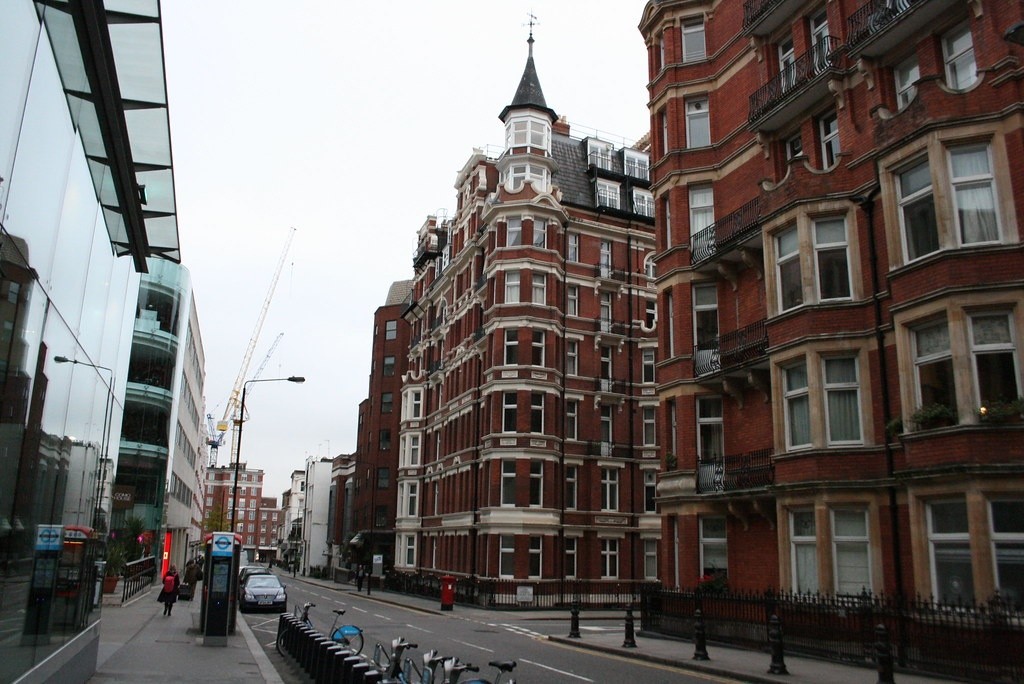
230 373 307 533
54 352 117 557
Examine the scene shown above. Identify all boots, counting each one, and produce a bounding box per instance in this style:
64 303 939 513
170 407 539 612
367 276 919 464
168 604 172 616
164 602 168 615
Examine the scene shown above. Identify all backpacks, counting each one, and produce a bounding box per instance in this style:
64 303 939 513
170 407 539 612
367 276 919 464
164 576 174 592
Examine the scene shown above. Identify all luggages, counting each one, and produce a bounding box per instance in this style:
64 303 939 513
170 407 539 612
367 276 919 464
178 585 190 601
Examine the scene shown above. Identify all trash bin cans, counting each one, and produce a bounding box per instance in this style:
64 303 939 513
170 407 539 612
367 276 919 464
441 576 457 611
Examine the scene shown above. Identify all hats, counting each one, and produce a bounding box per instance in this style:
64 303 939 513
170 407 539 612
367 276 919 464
170 563 176 571
190 559 194 563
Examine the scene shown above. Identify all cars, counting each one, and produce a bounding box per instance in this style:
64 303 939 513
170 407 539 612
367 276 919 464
237 566 289 613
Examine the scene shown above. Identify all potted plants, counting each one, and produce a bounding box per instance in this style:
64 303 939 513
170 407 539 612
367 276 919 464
101 545 126 594
883 396 1024 434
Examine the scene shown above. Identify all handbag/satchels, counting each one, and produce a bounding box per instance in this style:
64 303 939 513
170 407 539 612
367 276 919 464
197 570 203 581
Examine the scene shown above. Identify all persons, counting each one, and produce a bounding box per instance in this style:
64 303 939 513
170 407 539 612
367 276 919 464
157 563 181 618
185 554 205 602
355 564 366 591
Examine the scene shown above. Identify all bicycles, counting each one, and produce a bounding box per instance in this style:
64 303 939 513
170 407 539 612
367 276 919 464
276 602 365 658
373 637 516 684
